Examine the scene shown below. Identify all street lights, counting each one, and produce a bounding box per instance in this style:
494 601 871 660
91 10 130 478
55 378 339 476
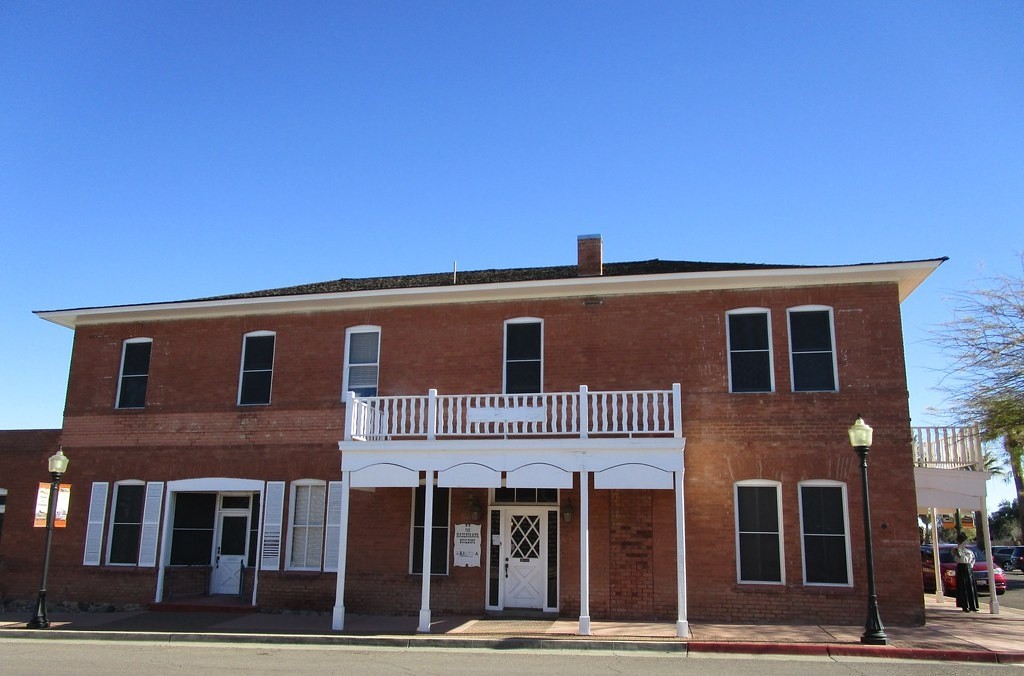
25 444 71 629
846 412 892 645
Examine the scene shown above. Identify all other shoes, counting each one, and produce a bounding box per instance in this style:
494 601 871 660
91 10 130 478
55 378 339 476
963 608 969 612
969 607 979 611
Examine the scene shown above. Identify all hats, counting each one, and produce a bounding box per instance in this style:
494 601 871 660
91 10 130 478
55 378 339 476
956 532 968 544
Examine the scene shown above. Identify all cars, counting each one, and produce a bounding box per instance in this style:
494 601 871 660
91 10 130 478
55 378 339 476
1011 546 1024 572
990 546 1017 571
921 543 1006 595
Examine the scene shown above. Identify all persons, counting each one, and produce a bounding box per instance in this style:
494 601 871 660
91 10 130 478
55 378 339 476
952 530 981 613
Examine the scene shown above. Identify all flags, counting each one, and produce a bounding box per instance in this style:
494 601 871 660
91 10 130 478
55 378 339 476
33 482 52 528
54 483 71 528
